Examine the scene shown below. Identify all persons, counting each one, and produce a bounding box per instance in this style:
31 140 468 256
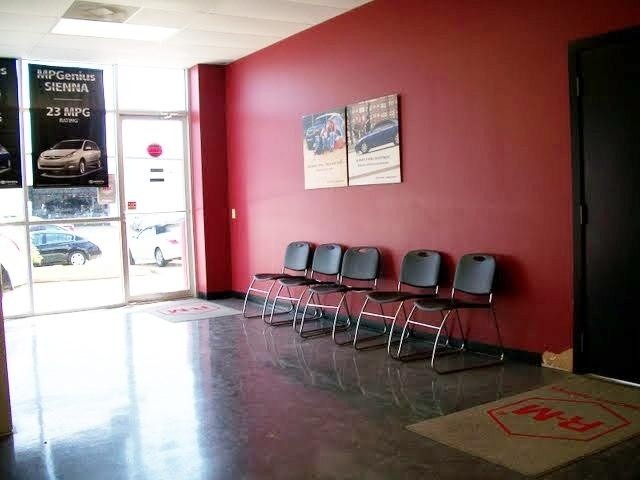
312 118 339 155
366 115 371 135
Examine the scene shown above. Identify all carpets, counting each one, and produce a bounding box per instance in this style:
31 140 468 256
406 375 639 479
150 300 242 322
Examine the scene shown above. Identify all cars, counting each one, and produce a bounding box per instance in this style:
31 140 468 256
352 115 399 156
37 136 103 176
128 217 183 269
0 212 104 295
0 141 14 179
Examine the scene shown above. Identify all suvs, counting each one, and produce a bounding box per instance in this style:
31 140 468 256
305 112 345 151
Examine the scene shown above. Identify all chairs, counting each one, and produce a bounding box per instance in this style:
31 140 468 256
353 249 449 359
270 244 351 333
243 242 318 324
299 247 387 345
397 254 505 375
